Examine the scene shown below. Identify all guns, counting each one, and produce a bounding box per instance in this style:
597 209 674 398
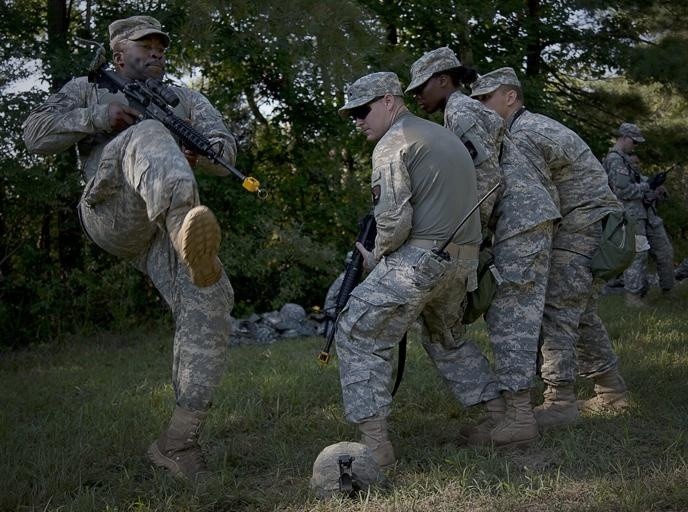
318 214 378 364
647 162 682 191
89 62 269 201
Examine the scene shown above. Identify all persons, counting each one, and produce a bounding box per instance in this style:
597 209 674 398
469 65 628 428
334 71 506 492
403 44 564 446
323 250 355 322
630 153 643 173
23 14 236 492
602 121 675 308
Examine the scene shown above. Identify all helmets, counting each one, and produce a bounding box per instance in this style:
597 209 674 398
236 319 258 337
255 327 274 345
310 440 381 495
280 303 306 322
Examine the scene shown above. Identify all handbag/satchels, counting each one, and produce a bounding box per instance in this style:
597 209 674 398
461 249 499 323
589 210 637 281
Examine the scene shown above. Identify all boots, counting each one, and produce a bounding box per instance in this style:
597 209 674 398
661 288 680 300
170 205 222 286
576 370 631 410
148 404 210 485
624 291 644 307
357 415 396 466
459 384 578 448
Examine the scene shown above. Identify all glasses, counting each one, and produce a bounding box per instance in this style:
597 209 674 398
353 97 384 120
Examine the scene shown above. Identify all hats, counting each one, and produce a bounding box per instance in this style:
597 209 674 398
109 16 170 52
620 123 645 142
403 47 462 94
469 67 521 97
338 72 404 121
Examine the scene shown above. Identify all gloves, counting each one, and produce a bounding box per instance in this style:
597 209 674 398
346 242 380 272
